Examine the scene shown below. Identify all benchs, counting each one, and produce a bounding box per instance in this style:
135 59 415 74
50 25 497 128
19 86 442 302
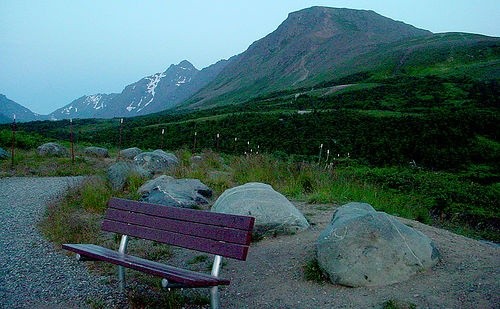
61 197 255 309
